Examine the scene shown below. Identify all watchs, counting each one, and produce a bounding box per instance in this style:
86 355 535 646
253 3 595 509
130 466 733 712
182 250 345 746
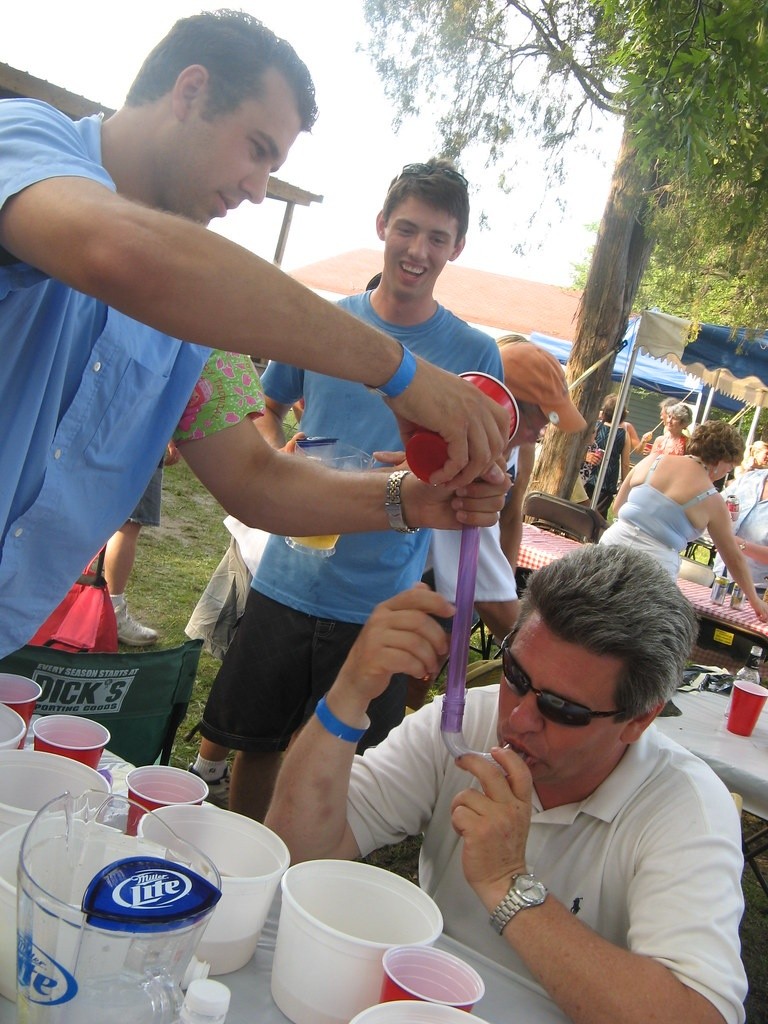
488 872 549 938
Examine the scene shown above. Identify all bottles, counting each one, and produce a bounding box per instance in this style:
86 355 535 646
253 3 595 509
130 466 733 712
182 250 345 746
725 646 762 720
171 980 231 1023
759 587 768 623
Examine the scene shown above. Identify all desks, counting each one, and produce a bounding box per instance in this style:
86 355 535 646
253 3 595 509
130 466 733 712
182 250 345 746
467 525 768 659
650 687 768 899
1 716 576 1024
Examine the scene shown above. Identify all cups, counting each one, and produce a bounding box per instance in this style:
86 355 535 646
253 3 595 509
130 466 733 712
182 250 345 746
272 860 443 1023
123 765 209 836
645 443 653 456
727 680 768 736
0 673 43 748
405 370 517 485
380 946 484 1012
1 749 112 835
348 1001 489 1024
0 816 133 1004
33 714 111 774
0 702 27 751
138 801 290 976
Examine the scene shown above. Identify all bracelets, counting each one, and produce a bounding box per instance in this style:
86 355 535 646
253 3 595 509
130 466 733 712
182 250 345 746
315 693 371 744
385 470 420 535
365 338 417 397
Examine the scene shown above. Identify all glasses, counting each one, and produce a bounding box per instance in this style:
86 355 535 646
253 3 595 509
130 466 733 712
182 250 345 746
395 162 468 196
520 408 539 443
501 628 625 727
727 463 734 473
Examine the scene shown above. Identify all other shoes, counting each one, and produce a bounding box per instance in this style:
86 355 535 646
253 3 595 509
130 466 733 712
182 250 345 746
186 762 231 795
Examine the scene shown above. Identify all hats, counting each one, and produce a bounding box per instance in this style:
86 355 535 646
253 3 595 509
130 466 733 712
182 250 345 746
494 335 587 433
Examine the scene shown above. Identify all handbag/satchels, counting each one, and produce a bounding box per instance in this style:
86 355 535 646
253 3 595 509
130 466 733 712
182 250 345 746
579 420 605 483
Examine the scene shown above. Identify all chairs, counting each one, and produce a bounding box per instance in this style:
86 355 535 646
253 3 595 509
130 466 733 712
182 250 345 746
524 491 600 544
0 639 204 768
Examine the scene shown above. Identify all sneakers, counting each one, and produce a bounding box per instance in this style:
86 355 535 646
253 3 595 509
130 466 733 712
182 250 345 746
113 598 157 646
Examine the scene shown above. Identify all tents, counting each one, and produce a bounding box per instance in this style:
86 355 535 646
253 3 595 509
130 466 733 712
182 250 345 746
587 310 768 514
531 331 745 413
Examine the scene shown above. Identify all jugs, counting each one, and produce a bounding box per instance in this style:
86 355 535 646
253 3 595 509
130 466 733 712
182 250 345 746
283 435 377 557
17 788 221 1024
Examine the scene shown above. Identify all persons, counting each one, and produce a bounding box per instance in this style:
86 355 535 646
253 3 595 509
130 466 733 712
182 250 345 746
0 11 514 662
264 545 750 1024
99 158 768 818
